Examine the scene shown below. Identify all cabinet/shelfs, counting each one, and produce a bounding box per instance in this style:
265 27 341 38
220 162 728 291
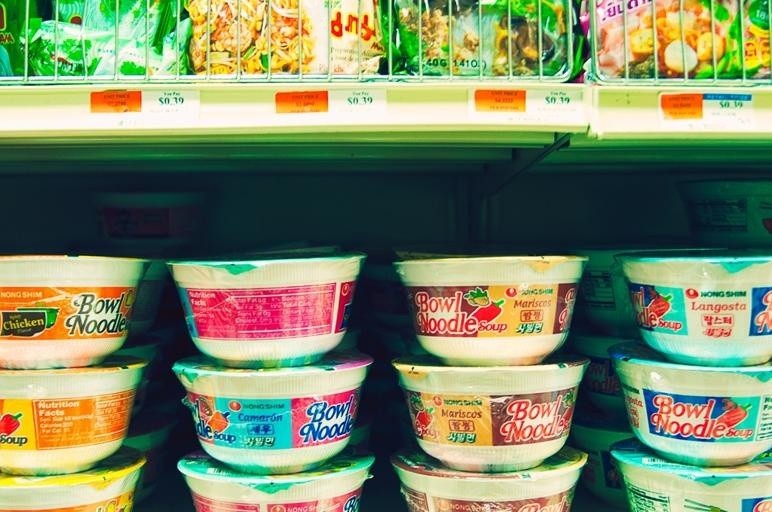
1 1 772 511
